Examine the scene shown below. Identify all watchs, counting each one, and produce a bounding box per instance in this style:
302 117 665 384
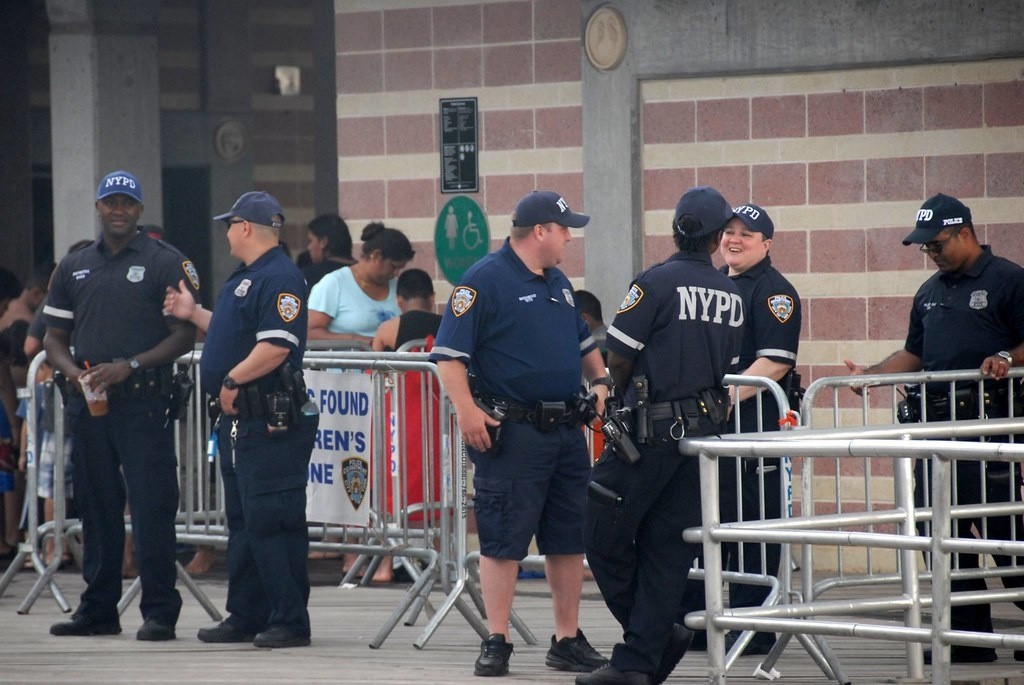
223 376 240 390
127 357 141 374
997 351 1012 365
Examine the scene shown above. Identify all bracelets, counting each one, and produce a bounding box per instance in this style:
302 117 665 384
592 377 612 391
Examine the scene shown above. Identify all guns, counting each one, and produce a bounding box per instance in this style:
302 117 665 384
472 397 505 455
53 370 75 406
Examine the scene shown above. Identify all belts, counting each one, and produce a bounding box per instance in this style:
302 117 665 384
502 406 572 422
647 400 702 421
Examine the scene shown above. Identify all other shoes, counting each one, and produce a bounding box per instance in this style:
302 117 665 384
922 647 993 665
724 630 776 657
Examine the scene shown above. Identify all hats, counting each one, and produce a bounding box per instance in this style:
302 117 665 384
212 191 286 227
674 186 732 237
902 193 971 246
728 204 775 238
511 190 590 228
97 171 142 202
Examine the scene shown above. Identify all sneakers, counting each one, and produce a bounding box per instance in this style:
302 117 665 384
197 621 256 643
652 622 694 685
50 612 122 636
136 617 175 640
546 629 609 672
253 627 312 647
474 633 514 677
575 661 654 685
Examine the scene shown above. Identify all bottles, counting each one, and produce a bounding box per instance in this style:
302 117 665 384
300 396 319 416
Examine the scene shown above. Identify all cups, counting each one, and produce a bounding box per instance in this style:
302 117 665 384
78 372 108 417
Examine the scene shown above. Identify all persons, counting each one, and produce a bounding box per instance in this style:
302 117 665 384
428 189 609 676
844 192 1024 664
574 290 610 352
184 214 442 581
164 190 320 646
574 186 746 685
685 203 801 655
0 239 94 568
41 170 201 641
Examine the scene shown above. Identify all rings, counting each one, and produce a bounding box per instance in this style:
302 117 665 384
999 362 1005 364
96 377 100 382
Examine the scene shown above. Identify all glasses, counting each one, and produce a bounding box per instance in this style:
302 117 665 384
920 227 961 254
227 218 245 228
